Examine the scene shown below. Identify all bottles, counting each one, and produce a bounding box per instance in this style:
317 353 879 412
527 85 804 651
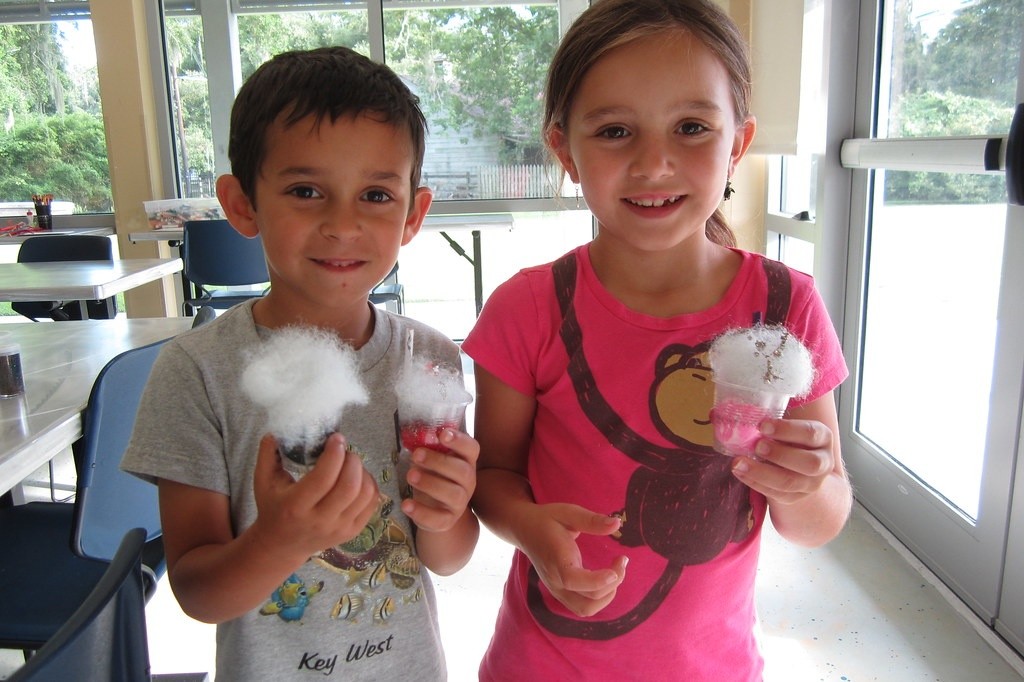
26 208 33 227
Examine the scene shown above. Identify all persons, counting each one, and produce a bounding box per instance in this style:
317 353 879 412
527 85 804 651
120 43 486 682
456 0 849 682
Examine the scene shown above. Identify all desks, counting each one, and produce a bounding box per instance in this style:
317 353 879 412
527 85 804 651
0 257 184 320
0 228 112 244
1 322 189 505
131 229 202 316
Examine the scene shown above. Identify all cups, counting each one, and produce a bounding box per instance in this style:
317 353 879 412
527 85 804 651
0 344 25 399
393 384 474 459
35 204 52 230
266 395 346 474
709 378 797 461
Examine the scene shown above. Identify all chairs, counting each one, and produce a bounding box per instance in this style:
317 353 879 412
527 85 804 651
0 307 216 660
367 262 405 316
185 220 271 309
12 235 117 321
0 527 151 682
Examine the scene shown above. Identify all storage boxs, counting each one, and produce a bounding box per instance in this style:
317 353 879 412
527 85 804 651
143 198 512 341
